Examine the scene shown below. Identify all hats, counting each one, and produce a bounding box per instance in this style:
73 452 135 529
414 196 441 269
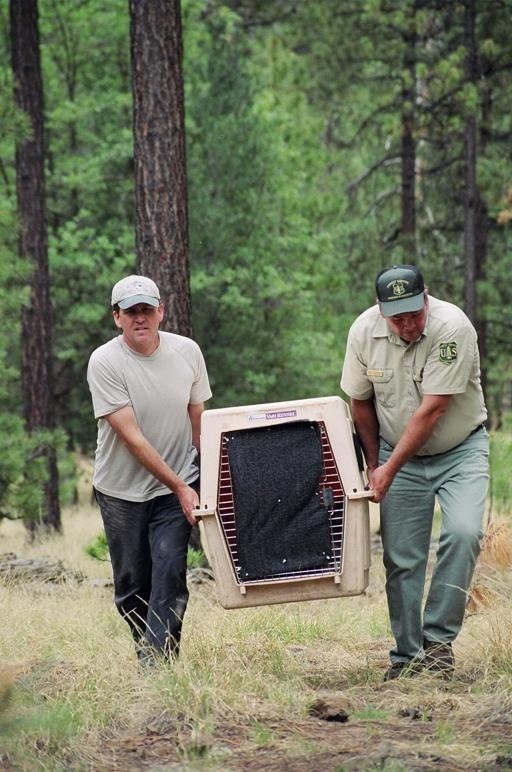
111 275 160 309
375 265 424 316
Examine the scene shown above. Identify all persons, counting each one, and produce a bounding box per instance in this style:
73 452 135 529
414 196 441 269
86 274 212 668
339 264 491 682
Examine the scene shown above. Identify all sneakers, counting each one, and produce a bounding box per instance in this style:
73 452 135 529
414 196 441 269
383 660 422 682
423 636 454 678
